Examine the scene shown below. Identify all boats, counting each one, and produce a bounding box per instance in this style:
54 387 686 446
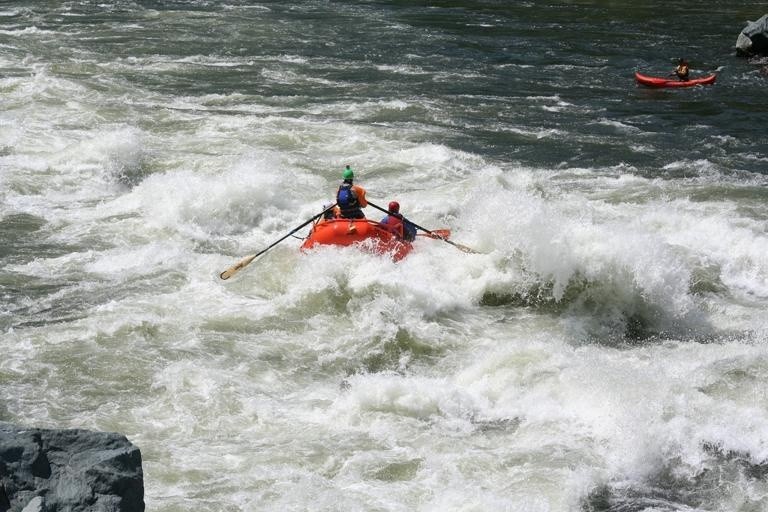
300 218 414 264
635 71 718 88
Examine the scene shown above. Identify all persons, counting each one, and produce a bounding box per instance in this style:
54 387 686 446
334 164 368 219
668 56 688 82
379 200 417 242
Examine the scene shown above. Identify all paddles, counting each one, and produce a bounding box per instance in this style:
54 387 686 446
219 202 338 280
368 202 484 255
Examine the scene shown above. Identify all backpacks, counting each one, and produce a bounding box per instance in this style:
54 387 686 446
337 184 357 209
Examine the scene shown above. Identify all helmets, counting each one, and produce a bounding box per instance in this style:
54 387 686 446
342 169 354 179
389 201 399 212
